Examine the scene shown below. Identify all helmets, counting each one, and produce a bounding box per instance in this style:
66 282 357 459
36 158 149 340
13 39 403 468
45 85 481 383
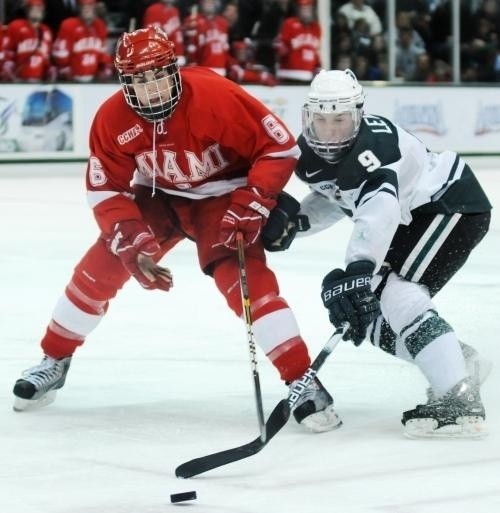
112 26 182 124
301 67 367 165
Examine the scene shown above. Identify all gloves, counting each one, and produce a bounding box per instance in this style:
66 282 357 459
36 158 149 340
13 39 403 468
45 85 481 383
319 260 382 346
219 188 278 253
104 221 174 292
262 190 311 252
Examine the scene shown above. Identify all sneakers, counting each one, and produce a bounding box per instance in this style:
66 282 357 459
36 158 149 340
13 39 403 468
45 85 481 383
285 373 335 425
400 377 486 423
13 354 72 401
426 342 492 398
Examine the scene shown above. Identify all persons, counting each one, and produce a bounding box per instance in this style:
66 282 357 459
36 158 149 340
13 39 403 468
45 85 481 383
12 25 344 435
261 67 492 442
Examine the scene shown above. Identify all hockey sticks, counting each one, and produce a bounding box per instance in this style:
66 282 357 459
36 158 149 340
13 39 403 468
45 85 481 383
174 264 389 477
236 230 267 442
